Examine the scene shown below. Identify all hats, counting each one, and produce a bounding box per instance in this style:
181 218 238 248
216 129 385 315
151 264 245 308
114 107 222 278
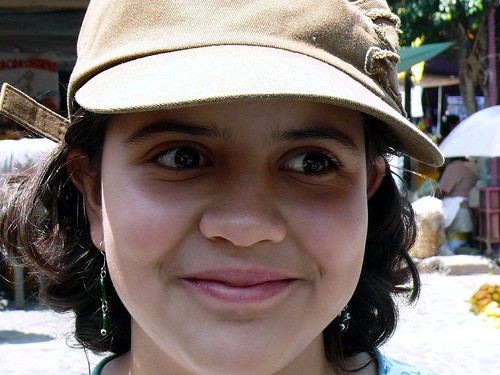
0 0 445 168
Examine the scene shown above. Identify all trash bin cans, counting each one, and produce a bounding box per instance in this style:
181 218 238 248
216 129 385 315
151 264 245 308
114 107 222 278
411 195 443 258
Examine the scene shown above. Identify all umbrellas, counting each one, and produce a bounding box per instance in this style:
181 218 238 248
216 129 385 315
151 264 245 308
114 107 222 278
438 105 500 157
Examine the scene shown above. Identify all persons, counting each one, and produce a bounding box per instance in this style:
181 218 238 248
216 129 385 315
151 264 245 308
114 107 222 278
1 2 444 375
417 114 480 233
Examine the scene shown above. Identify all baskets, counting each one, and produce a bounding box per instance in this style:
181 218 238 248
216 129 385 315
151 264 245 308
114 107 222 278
408 210 444 258
